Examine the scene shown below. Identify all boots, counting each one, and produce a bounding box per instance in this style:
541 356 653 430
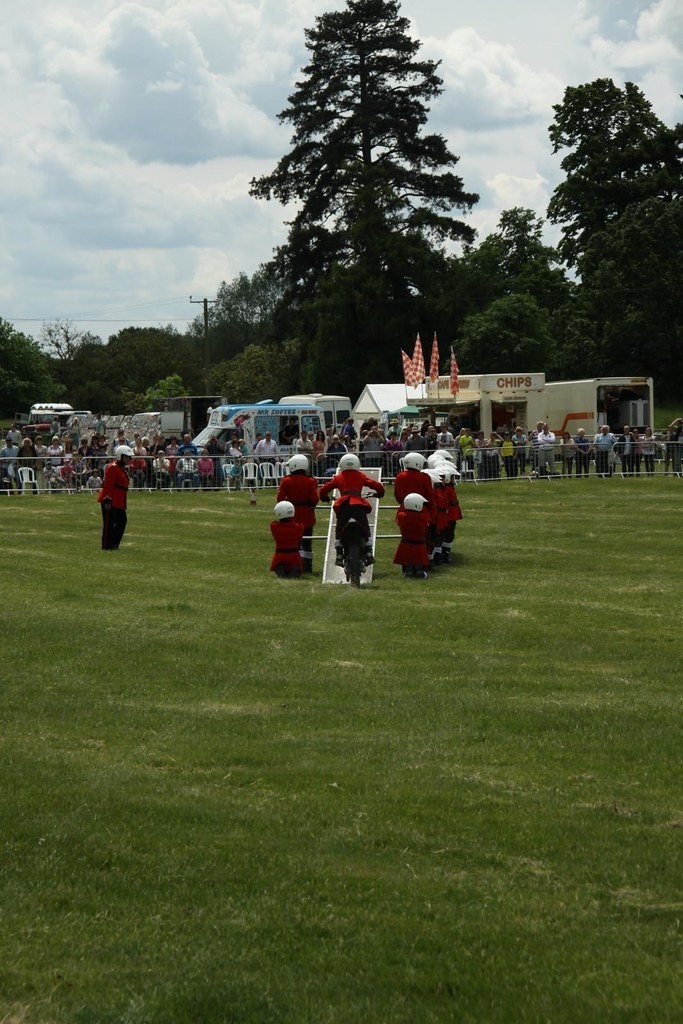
300 550 313 573
334 540 344 567
403 565 429 579
366 539 376 567
429 541 453 565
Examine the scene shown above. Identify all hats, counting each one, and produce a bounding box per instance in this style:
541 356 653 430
410 426 420 433
390 419 399 424
514 426 524 432
52 436 58 439
478 430 484 435
4 435 12 440
118 430 124 434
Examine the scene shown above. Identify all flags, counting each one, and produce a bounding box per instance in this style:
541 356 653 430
430 336 439 382
451 352 460 396
401 334 426 389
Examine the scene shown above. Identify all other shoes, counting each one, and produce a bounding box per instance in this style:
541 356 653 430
0 482 279 495
464 473 683 482
102 547 122 552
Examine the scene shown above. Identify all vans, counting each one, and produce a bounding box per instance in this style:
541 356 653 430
191 403 328 461
277 393 353 435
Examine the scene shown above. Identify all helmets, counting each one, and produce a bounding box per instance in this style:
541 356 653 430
338 453 361 470
404 493 429 511
115 445 135 461
402 450 462 489
284 454 309 473
274 500 295 520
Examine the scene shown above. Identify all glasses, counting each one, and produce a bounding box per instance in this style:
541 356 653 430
333 438 338 440
119 440 126 442
266 434 270 436
308 435 313 436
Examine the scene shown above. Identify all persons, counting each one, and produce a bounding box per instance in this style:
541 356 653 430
320 454 385 566
98 445 134 550
234 414 251 429
0 414 683 495
392 450 463 579
270 454 319 578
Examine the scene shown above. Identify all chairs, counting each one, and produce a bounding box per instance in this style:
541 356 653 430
459 460 477 485
155 461 290 492
18 467 39 495
42 466 72 494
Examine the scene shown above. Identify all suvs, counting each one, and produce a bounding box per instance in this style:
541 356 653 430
22 411 92 438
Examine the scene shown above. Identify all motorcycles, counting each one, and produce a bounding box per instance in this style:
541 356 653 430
323 491 384 587
